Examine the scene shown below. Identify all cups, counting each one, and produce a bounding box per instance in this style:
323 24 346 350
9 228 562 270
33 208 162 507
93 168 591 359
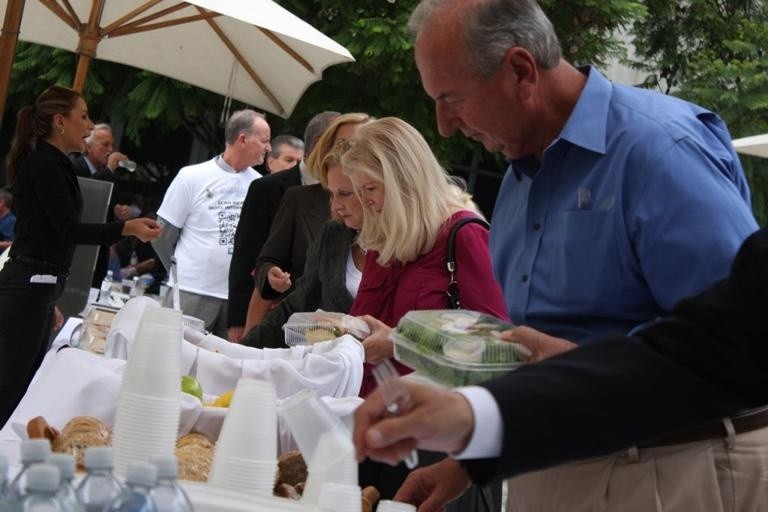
111 307 184 478
121 279 132 296
129 250 138 264
207 377 362 512
78 305 118 353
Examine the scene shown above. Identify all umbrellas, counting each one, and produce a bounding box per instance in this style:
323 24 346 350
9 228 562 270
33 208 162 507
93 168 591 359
0 0 362 121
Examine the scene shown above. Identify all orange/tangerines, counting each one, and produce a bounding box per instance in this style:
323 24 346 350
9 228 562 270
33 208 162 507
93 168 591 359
180 375 236 408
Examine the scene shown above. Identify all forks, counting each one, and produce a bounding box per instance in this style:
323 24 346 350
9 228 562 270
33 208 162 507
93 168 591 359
372 360 420 469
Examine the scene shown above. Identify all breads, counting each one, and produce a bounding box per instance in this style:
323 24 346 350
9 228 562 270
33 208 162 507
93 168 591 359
27 416 311 500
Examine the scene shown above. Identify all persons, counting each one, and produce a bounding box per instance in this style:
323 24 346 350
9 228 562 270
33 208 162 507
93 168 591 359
391 1 768 511
152 110 271 339
1 84 163 429
337 114 513 511
228 110 370 348
350 221 767 491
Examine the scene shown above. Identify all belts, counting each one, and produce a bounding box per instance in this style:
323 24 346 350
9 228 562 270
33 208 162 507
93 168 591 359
640 411 768 445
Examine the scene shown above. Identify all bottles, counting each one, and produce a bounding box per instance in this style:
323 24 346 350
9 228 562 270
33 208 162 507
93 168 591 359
117 153 136 172
0 435 194 512
97 269 113 306
